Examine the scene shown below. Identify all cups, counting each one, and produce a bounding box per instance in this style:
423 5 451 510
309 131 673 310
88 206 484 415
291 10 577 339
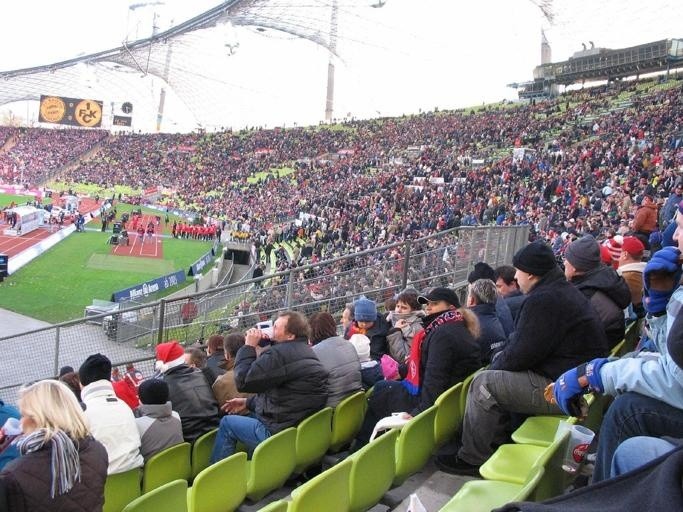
561 424 596 473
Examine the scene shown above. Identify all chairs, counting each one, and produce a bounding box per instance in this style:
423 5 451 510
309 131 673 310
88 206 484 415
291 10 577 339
443 100 524 117
461 367 486 421
190 428 219 484
396 406 438 486
191 130 256 169
255 501 290 512
254 232 308 275
511 393 595 447
198 181 248 198
582 75 680 121
245 158 298 186
331 391 368 455
90 157 121 164
543 120 578 138
347 428 397 512
298 407 331 477
141 441 190 497
187 452 247 512
288 458 353 511
366 384 374 400
438 465 545 512
609 338 627 357
435 382 462 458
118 479 188 512
46 181 144 201
625 320 638 338
489 145 513 161
302 120 362 141
134 277 281 354
478 430 571 501
246 426 297 501
103 466 141 512
158 195 238 225
532 93 590 117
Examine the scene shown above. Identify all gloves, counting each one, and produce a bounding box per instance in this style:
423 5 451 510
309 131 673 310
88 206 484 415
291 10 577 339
641 246 682 314
554 358 609 417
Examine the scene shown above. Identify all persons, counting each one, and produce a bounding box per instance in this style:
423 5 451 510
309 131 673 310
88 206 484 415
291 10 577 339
152 341 221 440
235 72 683 265
216 263 358 328
207 310 328 467
462 279 506 369
212 333 257 417
111 365 124 381
1 127 111 235
108 131 235 249
348 287 482 452
564 235 630 350
202 335 227 386
0 380 108 512
619 238 646 305
382 296 398 327
112 380 141 418
78 353 143 475
59 372 86 410
133 378 184 489
545 201 683 485
359 265 455 299
468 262 515 335
307 313 362 431
434 241 611 478
344 297 392 388
385 289 427 364
182 299 197 328
492 308 683 512
495 267 525 321
123 362 146 401
0 399 23 470
58 366 74 377
184 346 206 370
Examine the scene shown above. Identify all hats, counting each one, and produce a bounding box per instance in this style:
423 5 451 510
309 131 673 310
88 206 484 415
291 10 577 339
565 235 600 272
354 296 377 321
79 353 112 386
138 379 169 405
471 278 497 304
622 236 644 256
155 341 185 372
600 235 623 270
467 262 499 284
512 243 557 277
417 287 461 308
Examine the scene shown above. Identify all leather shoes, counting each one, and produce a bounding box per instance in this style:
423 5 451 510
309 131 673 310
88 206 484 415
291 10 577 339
434 455 478 475
